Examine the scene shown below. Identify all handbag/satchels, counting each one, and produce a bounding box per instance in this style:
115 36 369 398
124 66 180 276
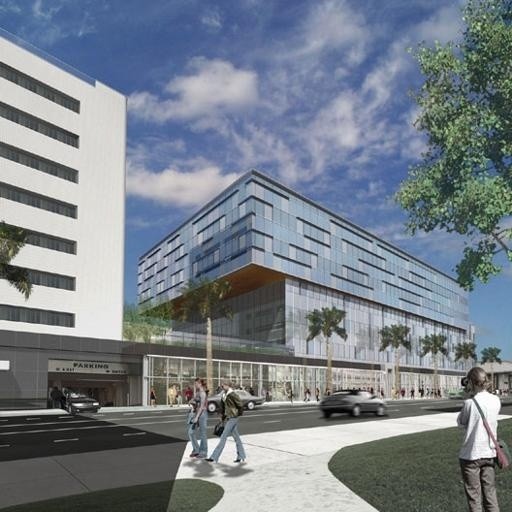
494 439 512 469
212 422 225 437
